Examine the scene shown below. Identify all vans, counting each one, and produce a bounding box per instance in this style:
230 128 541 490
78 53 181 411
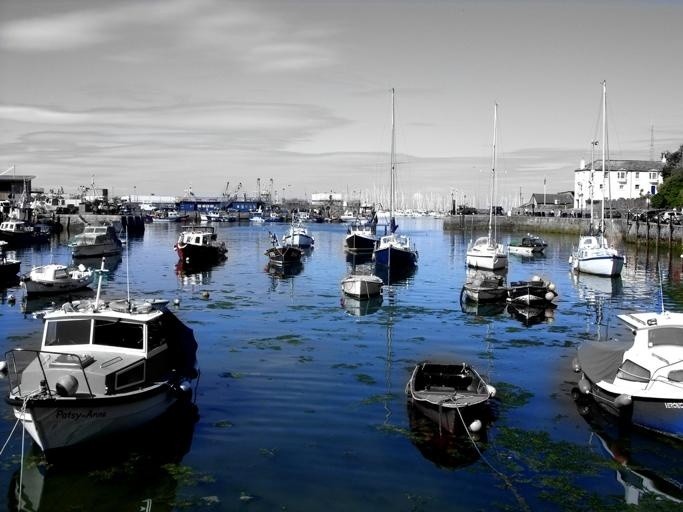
604 207 621 218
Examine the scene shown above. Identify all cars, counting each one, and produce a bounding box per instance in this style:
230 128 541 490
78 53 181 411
624 207 683 225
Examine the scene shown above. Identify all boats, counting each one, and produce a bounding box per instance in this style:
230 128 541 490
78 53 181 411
0 173 145 297
404 361 497 435
173 225 228 261
0 256 198 452
265 218 315 267
571 262 683 441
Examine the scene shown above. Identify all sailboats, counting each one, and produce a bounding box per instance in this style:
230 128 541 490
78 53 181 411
149 176 379 222
459 101 559 319
340 87 419 301
568 78 628 276
